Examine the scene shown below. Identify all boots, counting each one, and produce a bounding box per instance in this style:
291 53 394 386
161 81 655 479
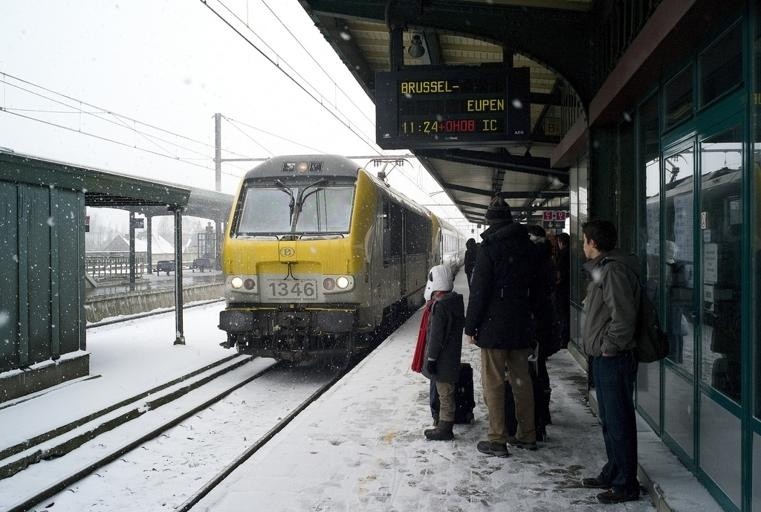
425 421 454 440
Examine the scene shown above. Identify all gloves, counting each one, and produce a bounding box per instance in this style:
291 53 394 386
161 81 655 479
427 361 437 374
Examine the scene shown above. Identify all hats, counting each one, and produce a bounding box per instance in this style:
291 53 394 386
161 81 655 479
423 265 455 300
485 191 514 224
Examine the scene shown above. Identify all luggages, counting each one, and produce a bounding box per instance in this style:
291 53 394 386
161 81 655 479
430 363 475 425
504 340 546 441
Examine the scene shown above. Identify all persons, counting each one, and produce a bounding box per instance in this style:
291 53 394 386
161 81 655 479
577 218 644 504
421 264 463 442
465 197 568 458
647 224 743 408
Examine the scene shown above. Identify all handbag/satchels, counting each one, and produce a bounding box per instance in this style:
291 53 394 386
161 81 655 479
637 291 670 363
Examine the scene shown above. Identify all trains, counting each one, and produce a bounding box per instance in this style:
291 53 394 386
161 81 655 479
646 169 742 324
218 154 468 369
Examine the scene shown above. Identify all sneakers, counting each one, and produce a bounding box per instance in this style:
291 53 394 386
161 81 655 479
477 441 508 458
582 472 611 488
597 488 640 504
507 436 537 451
